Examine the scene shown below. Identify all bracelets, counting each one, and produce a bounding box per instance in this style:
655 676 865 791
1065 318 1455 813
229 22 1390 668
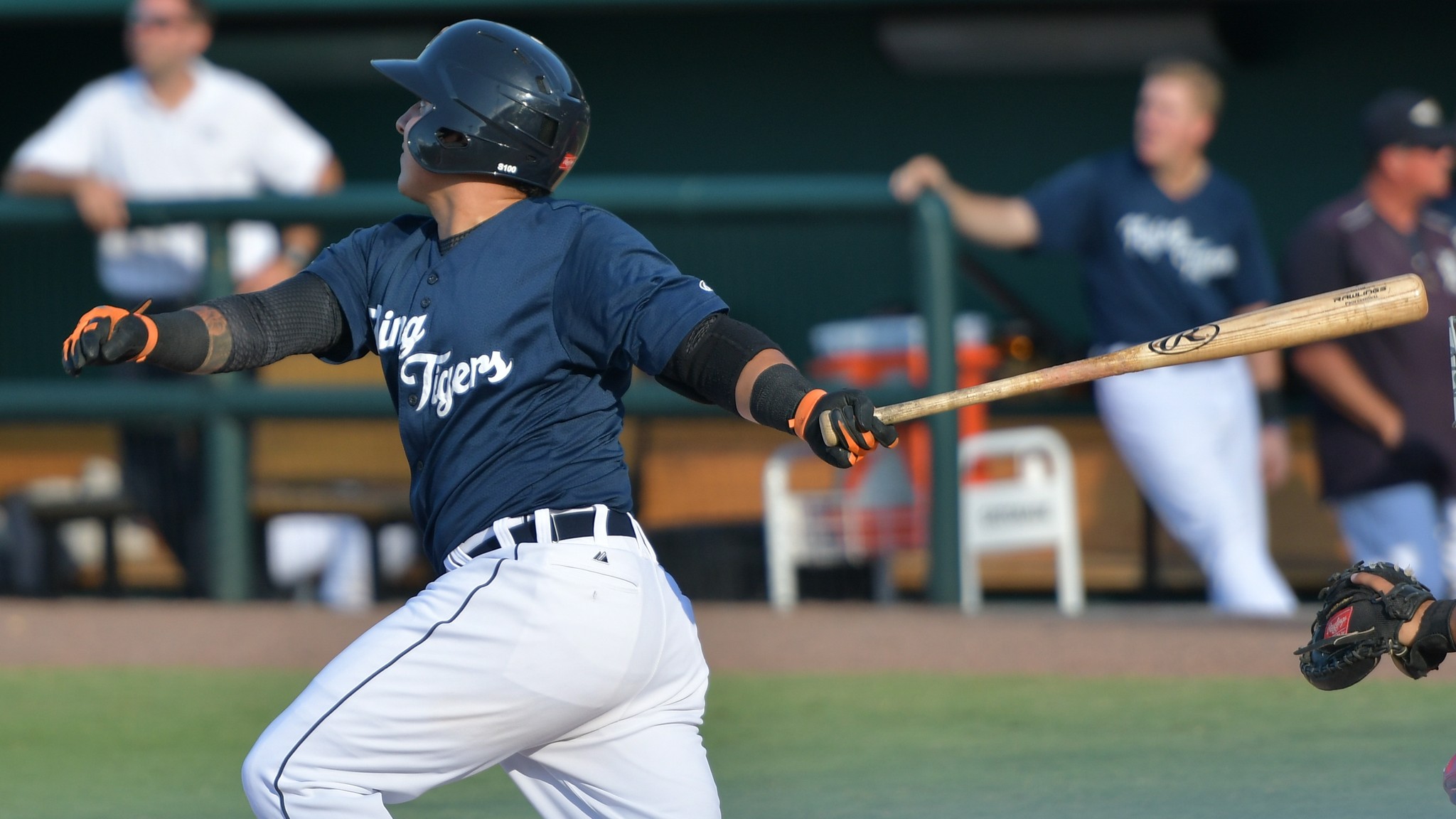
284 246 311 271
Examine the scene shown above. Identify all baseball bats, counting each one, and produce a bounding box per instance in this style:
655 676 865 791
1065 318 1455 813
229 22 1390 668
817 270 1430 450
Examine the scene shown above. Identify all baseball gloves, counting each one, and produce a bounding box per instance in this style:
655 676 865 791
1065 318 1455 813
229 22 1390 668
1297 561 1452 692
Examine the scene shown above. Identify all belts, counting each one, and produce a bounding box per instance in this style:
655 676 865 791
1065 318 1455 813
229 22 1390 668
466 509 636 560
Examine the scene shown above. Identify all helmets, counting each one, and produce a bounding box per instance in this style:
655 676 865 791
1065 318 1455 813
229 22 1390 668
369 20 591 197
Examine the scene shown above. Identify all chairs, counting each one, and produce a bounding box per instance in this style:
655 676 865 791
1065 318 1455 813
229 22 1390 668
761 428 1092 624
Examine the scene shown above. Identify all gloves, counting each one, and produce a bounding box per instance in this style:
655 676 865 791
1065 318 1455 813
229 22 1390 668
787 388 899 469
63 298 159 374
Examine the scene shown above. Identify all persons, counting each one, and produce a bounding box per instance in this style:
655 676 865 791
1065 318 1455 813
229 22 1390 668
7 0 344 604
62 18 900 819
1351 559 1456 805
888 57 1298 619
1288 85 1456 602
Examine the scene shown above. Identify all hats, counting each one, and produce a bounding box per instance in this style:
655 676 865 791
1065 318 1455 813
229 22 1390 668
1357 89 1456 148
